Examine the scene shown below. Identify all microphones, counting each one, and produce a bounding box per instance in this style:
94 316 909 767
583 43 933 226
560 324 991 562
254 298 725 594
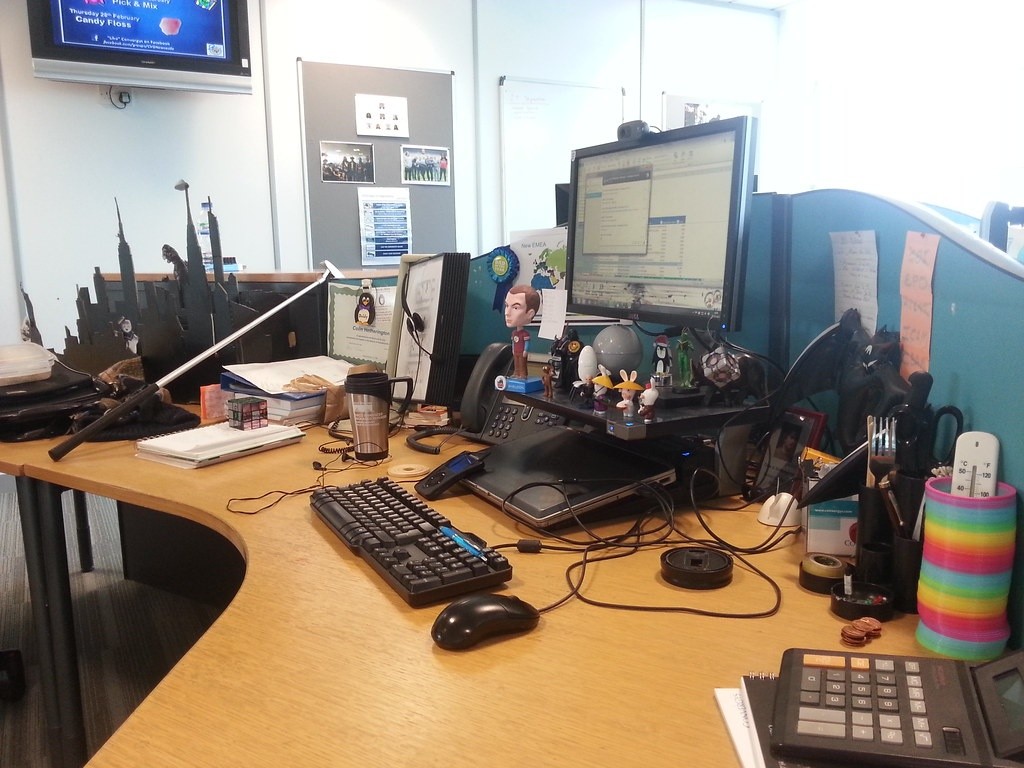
411 333 439 363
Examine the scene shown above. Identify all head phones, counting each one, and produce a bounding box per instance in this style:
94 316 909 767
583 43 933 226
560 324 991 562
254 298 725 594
401 273 425 335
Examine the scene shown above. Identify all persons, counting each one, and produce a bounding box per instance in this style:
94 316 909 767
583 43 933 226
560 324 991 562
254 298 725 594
774 426 801 462
541 366 557 398
322 156 374 182
504 284 540 379
404 149 447 182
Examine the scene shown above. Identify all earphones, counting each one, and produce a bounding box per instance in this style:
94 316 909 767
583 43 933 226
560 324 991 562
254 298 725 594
342 453 354 462
312 461 327 471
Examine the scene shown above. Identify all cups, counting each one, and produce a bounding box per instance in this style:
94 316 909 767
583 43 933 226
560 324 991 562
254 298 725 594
855 470 928 616
344 372 413 461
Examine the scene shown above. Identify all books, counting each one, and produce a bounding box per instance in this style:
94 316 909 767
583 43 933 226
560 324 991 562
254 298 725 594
714 672 858 768
134 421 306 469
220 362 350 430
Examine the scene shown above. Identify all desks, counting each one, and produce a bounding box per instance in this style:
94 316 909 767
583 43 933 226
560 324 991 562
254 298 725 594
1 403 1013 767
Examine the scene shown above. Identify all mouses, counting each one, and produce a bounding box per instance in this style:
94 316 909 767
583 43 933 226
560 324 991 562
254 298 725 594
431 592 541 650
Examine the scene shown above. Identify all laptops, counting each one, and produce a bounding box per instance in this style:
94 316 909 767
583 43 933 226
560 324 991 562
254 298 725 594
458 425 676 528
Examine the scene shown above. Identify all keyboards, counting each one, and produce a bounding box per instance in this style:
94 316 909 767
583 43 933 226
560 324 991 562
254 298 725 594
310 476 513 608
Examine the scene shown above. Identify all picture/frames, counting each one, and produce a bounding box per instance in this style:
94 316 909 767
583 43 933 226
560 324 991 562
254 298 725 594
752 405 828 505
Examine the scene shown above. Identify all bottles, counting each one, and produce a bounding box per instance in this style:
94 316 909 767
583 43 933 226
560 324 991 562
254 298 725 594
197 202 218 275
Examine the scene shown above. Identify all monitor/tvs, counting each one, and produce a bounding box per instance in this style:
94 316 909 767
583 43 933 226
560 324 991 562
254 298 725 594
27 0 254 95
564 115 758 408
